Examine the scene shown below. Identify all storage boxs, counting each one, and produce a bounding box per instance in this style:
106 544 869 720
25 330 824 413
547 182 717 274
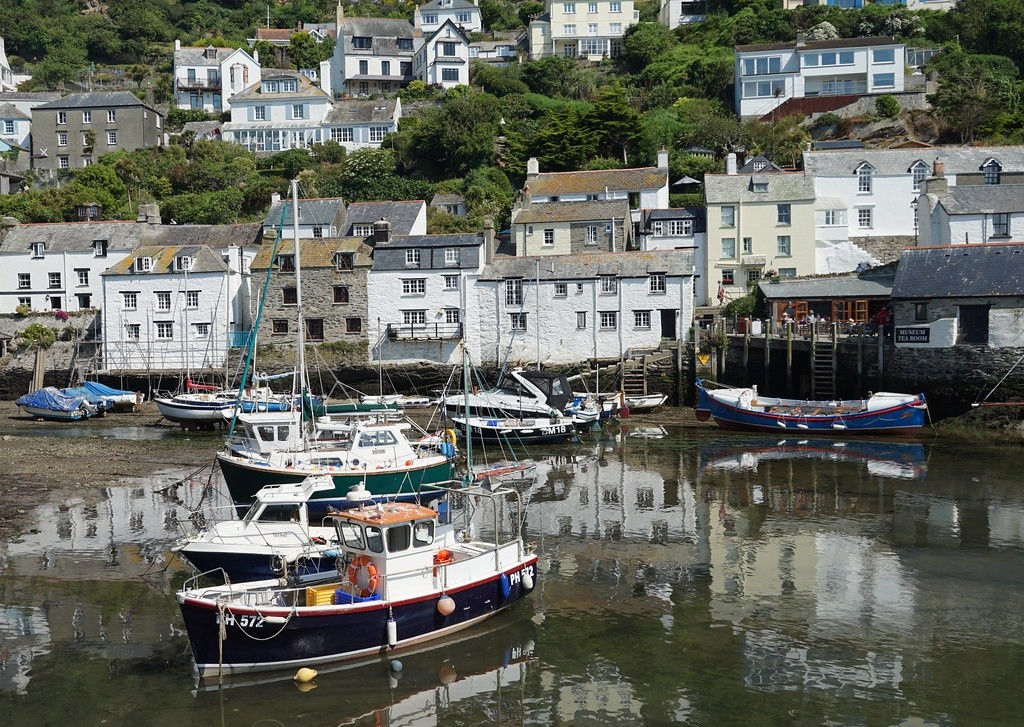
305 584 381 606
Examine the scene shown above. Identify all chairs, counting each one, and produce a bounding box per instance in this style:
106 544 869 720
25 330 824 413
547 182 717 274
781 320 828 335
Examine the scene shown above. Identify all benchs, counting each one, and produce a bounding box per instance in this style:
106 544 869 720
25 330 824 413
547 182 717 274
789 406 800 414
835 408 844 414
811 407 822 416
769 406 778 413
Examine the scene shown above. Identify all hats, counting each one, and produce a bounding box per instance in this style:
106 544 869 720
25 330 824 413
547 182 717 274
783 313 787 316
809 310 814 313
882 307 886 310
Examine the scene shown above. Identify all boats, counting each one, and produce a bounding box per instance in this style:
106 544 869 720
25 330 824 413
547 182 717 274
154 176 668 583
174 482 539 679
700 435 928 483
193 599 541 727
14 379 146 422
694 376 928 437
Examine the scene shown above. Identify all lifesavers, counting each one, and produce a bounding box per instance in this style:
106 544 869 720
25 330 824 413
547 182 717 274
349 557 379 599
444 428 457 448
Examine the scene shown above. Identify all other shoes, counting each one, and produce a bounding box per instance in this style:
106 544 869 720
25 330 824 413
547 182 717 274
780 335 784 338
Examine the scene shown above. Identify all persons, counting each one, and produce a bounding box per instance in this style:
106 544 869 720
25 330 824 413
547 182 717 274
780 307 892 339
